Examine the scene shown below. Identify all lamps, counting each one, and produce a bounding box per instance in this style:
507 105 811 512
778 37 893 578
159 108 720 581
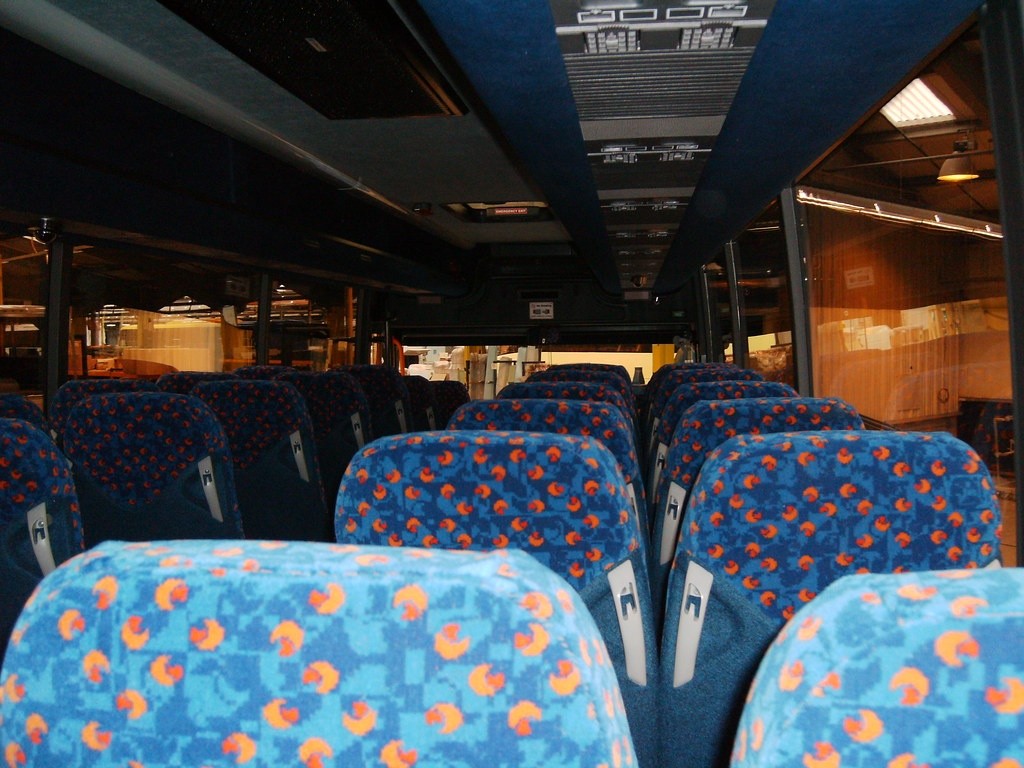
937 150 980 181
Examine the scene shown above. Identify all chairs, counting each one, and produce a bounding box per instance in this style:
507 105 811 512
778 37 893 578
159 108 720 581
0 359 1024 768
